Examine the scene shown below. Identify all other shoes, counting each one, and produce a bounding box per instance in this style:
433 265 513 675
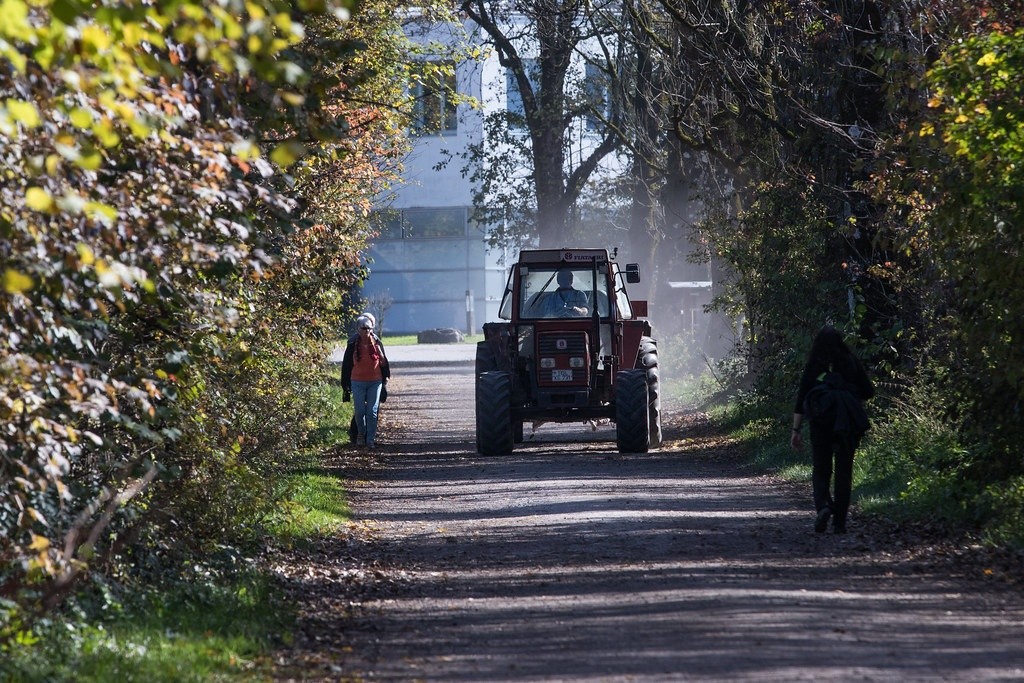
815 508 831 533
368 444 375 449
832 516 847 534
357 433 366 446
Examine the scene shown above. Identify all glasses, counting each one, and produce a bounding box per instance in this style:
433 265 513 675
360 326 372 330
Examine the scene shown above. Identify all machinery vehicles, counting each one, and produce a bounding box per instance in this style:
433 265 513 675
474 246 662 456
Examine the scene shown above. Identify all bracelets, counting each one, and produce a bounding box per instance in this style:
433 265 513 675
792 428 799 432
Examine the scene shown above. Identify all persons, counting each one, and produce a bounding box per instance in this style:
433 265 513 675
791 323 877 534
341 312 390 448
543 269 588 318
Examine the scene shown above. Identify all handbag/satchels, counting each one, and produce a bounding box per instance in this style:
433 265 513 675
834 345 875 399
379 355 390 380
